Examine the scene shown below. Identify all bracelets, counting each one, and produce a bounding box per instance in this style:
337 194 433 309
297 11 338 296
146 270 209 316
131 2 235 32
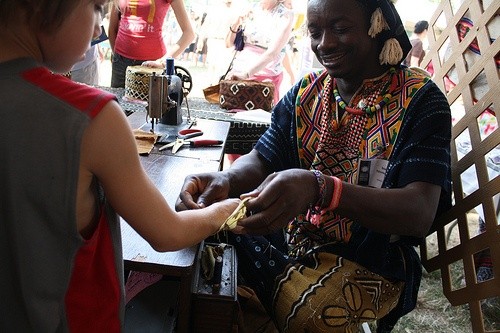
306 170 342 229
230 24 243 34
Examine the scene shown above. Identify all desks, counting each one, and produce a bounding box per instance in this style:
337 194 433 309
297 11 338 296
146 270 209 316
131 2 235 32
119 112 233 333
88 84 276 154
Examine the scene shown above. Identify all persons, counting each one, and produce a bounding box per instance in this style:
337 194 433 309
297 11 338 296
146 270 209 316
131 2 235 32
108 0 195 88
168 10 210 68
403 20 429 68
175 0 452 333
225 0 296 107
0 0 252 333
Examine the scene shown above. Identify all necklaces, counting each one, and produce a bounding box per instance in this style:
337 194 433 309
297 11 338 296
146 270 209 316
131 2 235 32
332 65 398 114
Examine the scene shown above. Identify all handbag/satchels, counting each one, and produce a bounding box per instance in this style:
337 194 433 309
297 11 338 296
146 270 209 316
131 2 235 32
203 78 220 104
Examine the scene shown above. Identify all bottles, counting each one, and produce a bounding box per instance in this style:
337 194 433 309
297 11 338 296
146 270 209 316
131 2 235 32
161 58 182 125
